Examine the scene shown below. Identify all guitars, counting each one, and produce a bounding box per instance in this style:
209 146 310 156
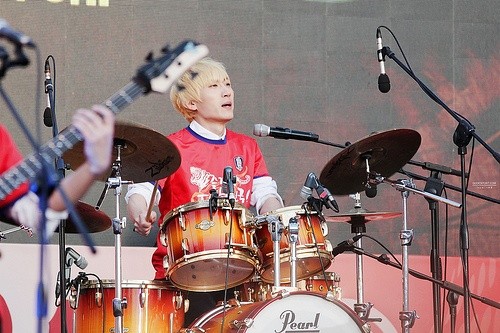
0 40 210 210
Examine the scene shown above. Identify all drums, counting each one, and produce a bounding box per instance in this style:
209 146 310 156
72 278 185 333
253 204 334 284
179 289 373 333
158 198 259 292
239 272 342 305
52 201 111 234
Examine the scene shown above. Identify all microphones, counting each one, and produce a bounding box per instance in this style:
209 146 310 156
366 185 378 198
299 171 339 213
252 124 320 143
375 27 390 93
223 166 236 207
43 60 57 128
66 247 89 270
0 19 37 50
330 235 362 259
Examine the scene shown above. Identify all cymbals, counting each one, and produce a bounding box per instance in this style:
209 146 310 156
61 122 181 184
325 211 403 221
319 129 422 196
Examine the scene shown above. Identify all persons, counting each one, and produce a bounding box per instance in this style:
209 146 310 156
0 104 115 333
125 58 285 327
